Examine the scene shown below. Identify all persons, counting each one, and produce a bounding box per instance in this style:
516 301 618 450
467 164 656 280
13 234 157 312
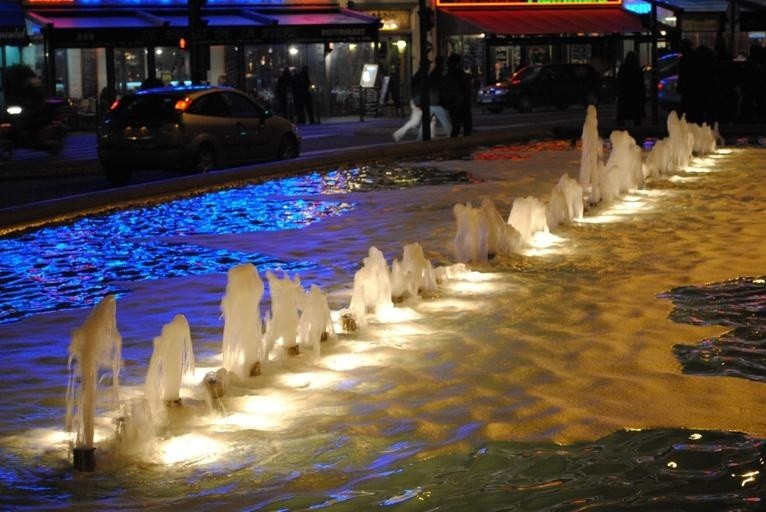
287 66 317 125
216 75 231 86
389 54 474 143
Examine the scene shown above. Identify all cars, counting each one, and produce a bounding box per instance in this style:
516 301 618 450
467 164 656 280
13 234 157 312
477 64 601 114
97 85 302 182
642 53 689 112
0 68 80 153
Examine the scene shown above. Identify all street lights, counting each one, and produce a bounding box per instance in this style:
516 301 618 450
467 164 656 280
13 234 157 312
397 39 408 119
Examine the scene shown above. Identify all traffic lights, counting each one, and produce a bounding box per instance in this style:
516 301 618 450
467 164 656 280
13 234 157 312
177 35 190 50
